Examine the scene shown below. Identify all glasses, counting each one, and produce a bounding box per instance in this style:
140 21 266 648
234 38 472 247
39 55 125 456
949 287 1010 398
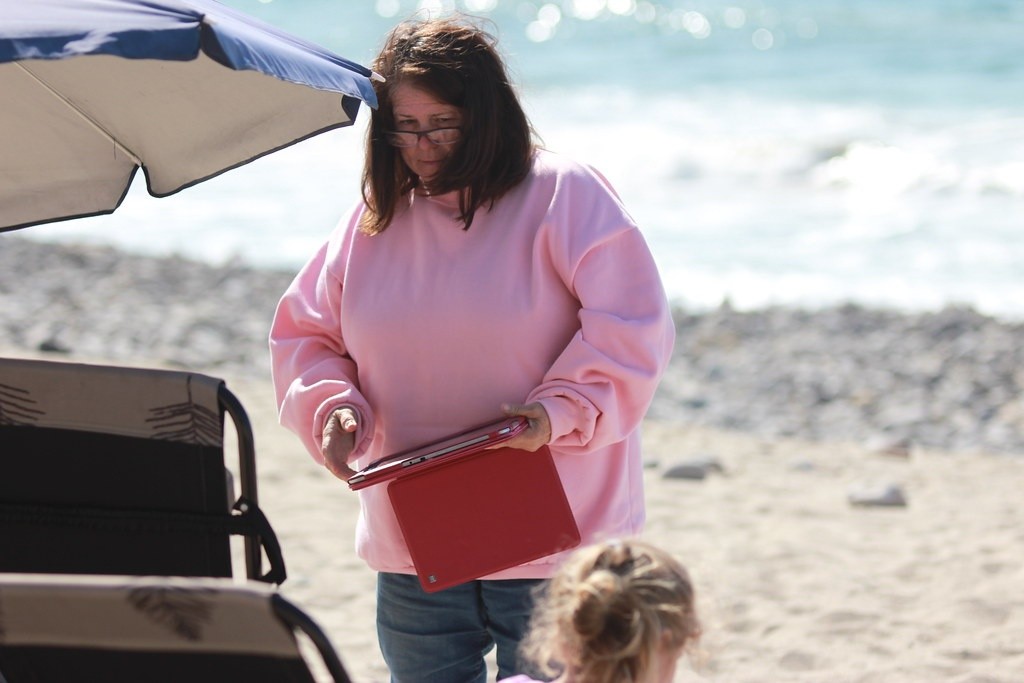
382 122 464 146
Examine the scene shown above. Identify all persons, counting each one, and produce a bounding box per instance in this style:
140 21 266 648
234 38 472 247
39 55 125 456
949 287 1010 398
269 17 678 683
494 534 706 683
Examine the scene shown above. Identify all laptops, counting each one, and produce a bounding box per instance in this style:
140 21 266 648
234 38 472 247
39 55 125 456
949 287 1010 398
349 417 529 491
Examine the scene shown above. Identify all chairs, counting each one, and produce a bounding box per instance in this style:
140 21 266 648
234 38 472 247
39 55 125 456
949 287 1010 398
0 571 352 683
0 358 286 586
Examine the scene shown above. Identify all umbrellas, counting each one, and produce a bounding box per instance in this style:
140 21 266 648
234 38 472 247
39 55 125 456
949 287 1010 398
0 0 389 233
